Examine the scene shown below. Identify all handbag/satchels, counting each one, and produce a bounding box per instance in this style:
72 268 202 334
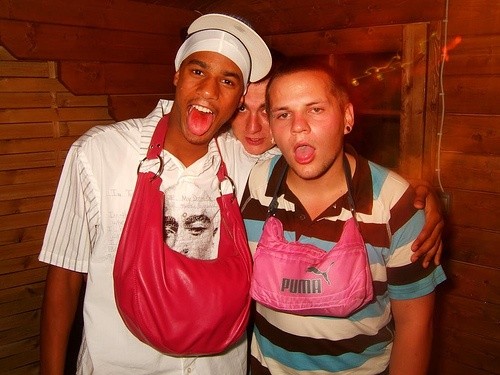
116 111 254 357
248 152 374 318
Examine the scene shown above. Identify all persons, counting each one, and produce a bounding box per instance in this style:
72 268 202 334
231 56 447 375
33 10 446 375
221 43 299 159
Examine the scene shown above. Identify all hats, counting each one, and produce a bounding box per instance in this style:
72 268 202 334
174 13 272 96
